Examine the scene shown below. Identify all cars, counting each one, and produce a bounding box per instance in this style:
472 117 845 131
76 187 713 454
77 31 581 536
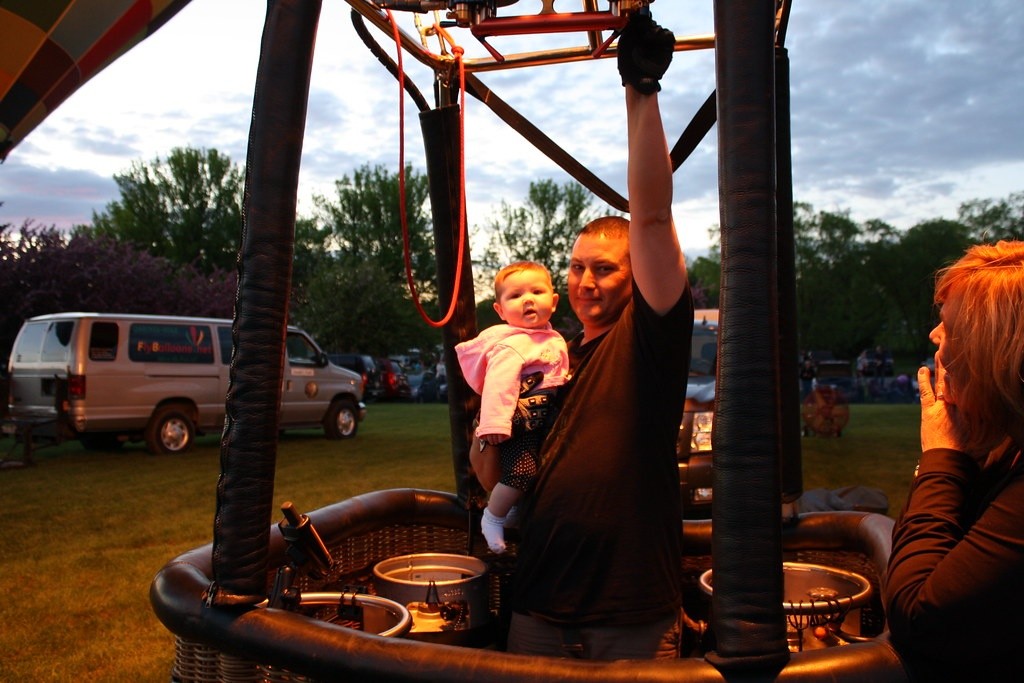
811 359 863 403
855 348 896 375
380 346 447 401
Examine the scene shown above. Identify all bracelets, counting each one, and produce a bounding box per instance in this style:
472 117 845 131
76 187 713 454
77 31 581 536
914 465 919 477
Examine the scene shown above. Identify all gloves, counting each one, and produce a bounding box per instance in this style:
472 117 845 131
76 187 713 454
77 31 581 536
472 371 554 446
617 8 676 95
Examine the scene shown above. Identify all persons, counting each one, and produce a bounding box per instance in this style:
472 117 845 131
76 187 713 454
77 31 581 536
801 352 829 437
884 240 1024 683
857 343 915 404
469 8 692 661
454 261 570 556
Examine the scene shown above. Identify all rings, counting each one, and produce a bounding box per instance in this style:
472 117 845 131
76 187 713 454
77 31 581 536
937 392 944 399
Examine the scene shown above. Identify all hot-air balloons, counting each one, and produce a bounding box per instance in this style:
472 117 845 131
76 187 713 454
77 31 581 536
0 0 920 683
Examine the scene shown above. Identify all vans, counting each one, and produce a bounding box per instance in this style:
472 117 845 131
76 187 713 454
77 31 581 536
674 308 724 511
1 310 366 458
326 353 377 402
